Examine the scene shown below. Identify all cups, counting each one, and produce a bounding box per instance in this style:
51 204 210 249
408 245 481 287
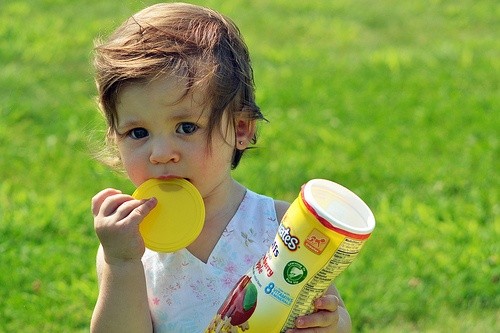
204 172 376 331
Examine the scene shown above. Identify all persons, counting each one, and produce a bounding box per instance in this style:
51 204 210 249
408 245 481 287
88 3 353 333
